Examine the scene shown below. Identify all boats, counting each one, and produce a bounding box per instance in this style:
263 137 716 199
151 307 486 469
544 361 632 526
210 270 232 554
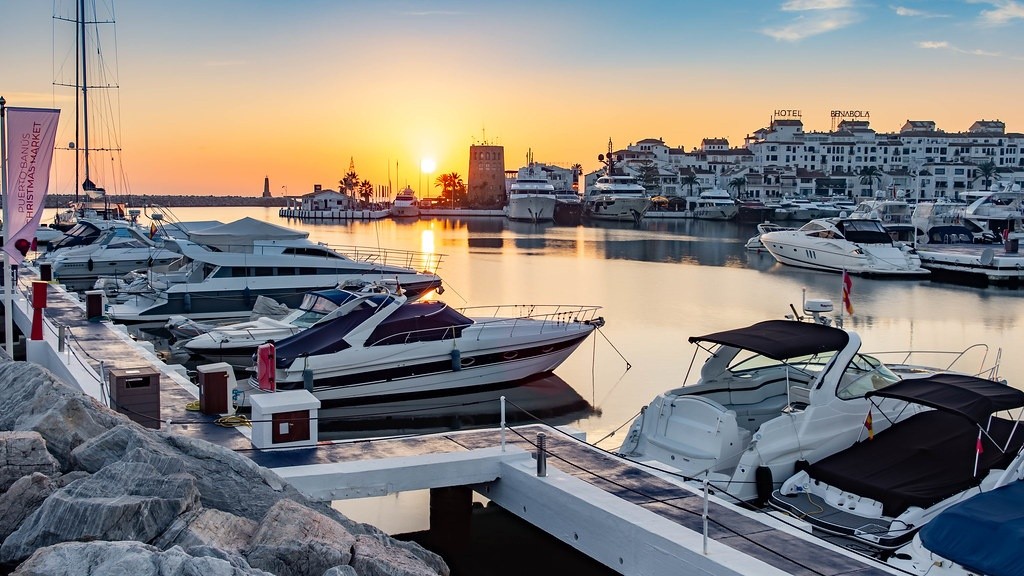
619 294 1023 576
169 280 406 368
693 186 740 221
234 280 603 424
651 196 669 207
581 137 653 223
504 146 555 222
390 185 420 217
552 179 581 212
668 197 687 208
741 181 1024 279
30 211 447 341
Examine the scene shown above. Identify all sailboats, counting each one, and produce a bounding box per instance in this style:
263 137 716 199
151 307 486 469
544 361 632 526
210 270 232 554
18 0 222 258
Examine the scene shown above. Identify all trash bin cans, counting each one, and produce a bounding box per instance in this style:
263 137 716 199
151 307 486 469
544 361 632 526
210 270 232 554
109 364 163 431
40 261 52 281
11 264 18 281
85 289 105 319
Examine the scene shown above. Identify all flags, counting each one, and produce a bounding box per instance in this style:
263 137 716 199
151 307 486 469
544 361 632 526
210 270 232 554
865 411 874 439
149 223 157 238
396 282 402 295
843 273 854 314
0 107 60 264
